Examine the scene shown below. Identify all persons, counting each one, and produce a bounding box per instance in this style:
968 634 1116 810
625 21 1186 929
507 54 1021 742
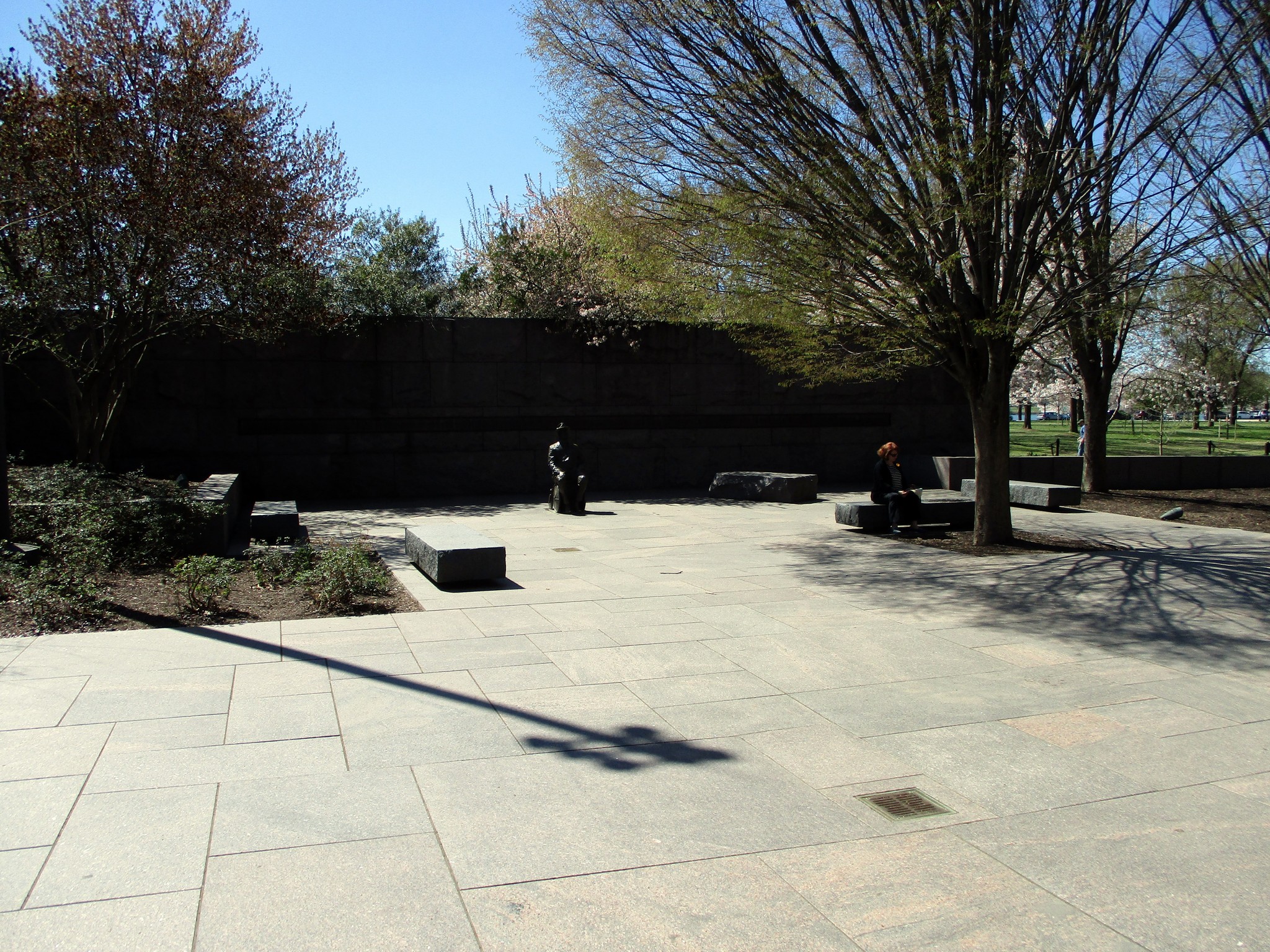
1076 419 1085 456
1258 410 1262 422
871 442 921 535
548 422 587 517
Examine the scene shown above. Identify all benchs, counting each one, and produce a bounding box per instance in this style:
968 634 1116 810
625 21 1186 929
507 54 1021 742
708 471 818 504
960 478 1081 512
405 527 506 591
835 498 976 531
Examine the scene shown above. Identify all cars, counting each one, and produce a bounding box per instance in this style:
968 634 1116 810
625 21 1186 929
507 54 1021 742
1107 409 1132 420
1252 409 1270 420
1009 415 1013 421
1133 410 1149 420
1165 411 1181 420
1203 410 1230 420
1036 411 1065 420
1063 412 1070 421
1228 411 1254 419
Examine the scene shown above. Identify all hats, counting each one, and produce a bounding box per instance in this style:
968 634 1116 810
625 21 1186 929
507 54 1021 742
1077 419 1084 423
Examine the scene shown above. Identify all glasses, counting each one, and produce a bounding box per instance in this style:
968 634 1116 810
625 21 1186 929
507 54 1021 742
889 453 898 457
